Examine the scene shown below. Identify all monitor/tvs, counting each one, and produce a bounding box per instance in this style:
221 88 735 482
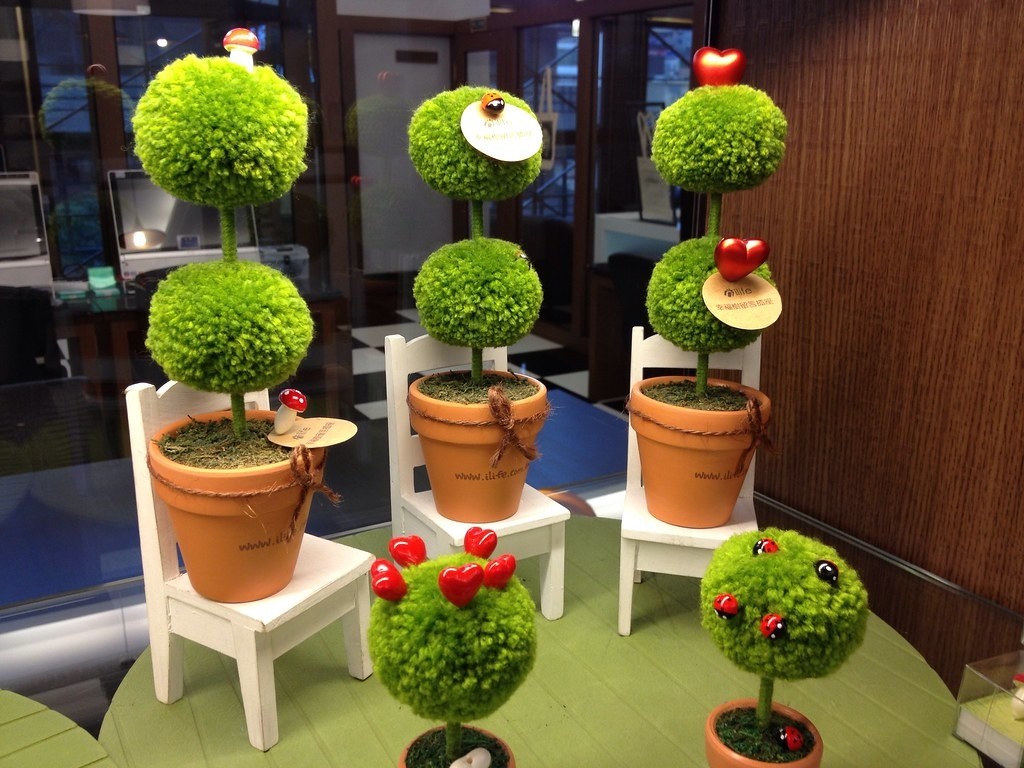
0 171 54 287
107 169 261 295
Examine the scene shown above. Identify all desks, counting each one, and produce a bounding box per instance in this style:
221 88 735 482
585 212 684 401
92 516 984 767
36 286 340 456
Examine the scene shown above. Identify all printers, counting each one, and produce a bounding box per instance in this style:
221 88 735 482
260 242 311 280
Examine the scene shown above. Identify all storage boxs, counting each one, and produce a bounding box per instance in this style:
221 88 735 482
952 650 1024 768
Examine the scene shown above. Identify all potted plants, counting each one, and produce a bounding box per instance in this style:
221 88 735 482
410 83 548 522
700 522 868 768
368 530 537 768
148 23 327 605
626 48 787 530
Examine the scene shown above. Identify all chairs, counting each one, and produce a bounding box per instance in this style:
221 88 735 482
616 324 762 634
384 336 572 618
127 381 373 750
